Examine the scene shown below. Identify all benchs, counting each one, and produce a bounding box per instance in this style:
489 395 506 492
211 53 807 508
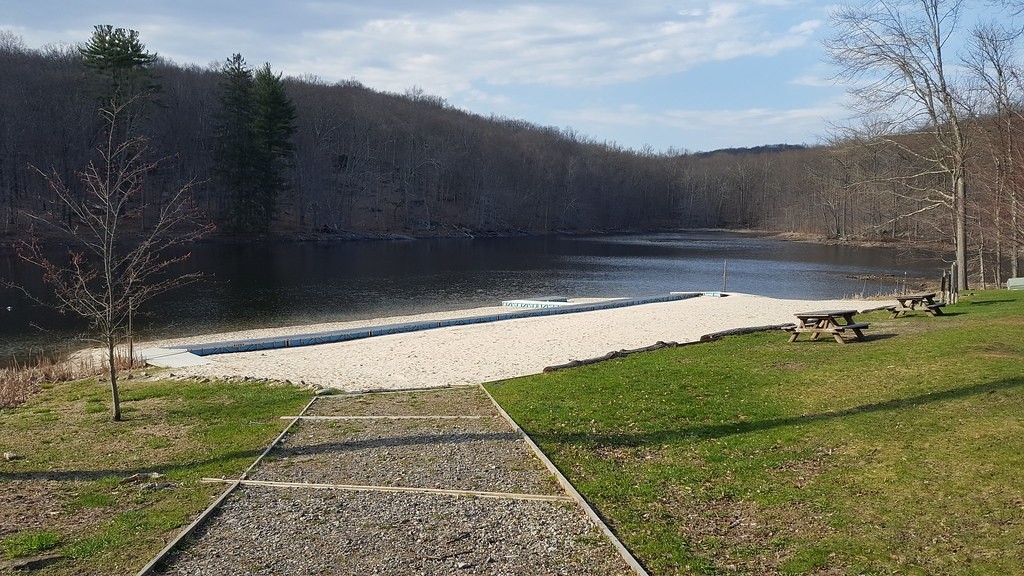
926 302 946 310
781 323 815 332
886 305 915 311
834 322 871 333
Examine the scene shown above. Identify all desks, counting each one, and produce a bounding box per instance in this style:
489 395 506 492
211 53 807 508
788 310 864 344
889 293 944 319
